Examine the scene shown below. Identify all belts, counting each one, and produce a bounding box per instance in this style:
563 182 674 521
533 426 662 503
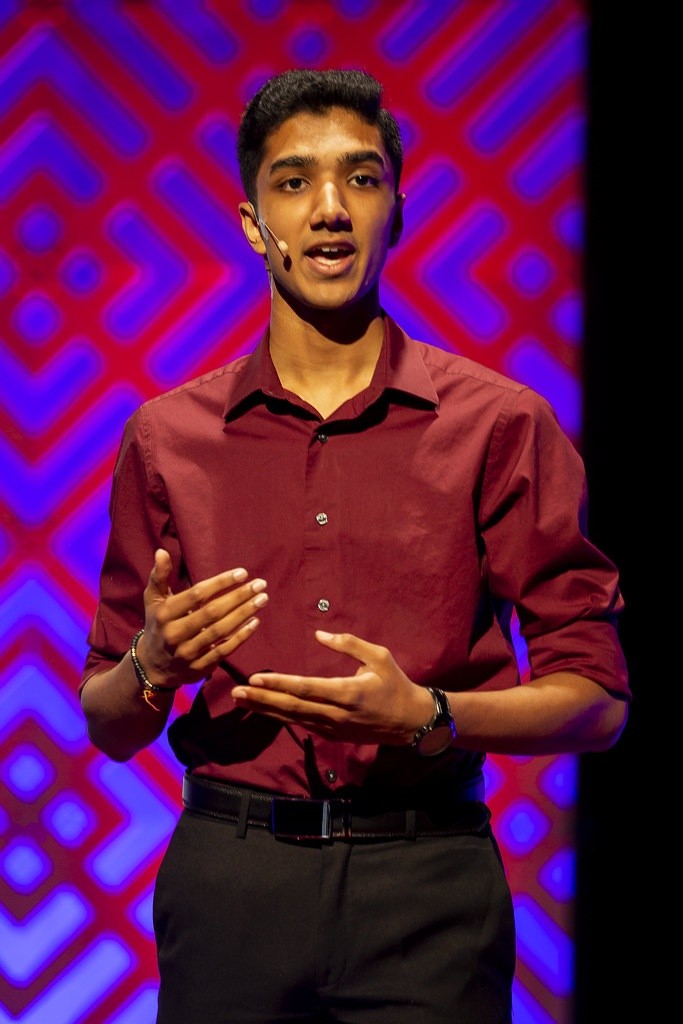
181 769 491 842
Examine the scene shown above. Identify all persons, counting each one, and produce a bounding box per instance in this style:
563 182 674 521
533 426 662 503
77 69 636 1024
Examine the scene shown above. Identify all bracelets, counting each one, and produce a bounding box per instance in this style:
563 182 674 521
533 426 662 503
131 629 177 711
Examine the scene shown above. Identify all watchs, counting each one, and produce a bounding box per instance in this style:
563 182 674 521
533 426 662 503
408 688 457 755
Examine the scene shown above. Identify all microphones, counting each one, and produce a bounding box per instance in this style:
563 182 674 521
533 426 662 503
259 217 289 253
